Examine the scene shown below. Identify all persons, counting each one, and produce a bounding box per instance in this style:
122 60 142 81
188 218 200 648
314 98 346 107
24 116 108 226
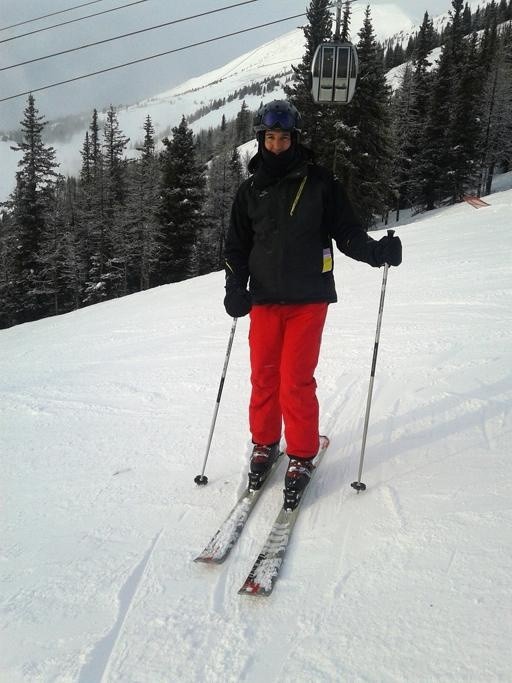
223 101 401 514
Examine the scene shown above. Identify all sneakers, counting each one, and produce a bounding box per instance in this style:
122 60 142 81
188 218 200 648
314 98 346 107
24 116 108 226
250 442 279 472
285 459 313 492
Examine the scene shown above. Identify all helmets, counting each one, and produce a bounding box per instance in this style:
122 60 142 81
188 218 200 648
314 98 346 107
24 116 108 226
254 100 302 134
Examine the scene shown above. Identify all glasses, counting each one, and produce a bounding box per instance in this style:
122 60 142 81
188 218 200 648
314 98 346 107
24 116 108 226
253 112 304 129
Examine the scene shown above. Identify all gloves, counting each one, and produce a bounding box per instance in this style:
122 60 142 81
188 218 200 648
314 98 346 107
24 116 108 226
380 237 402 266
225 286 251 317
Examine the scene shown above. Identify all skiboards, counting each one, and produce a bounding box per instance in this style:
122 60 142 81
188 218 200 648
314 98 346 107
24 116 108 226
192 434 329 596
